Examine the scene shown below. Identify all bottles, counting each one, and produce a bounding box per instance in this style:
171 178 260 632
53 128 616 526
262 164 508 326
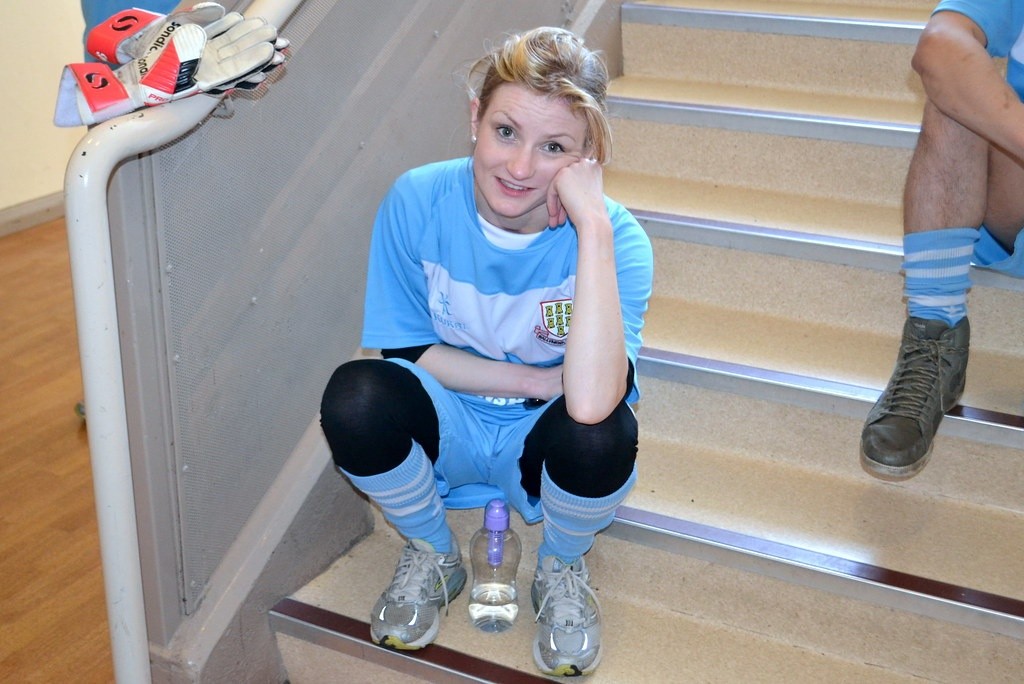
468 498 521 635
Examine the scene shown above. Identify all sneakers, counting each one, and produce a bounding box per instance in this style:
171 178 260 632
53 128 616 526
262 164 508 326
859 315 971 482
370 527 468 650
530 556 604 678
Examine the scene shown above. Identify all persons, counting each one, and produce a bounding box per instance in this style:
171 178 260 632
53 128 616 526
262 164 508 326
74 0 180 419
860 0 1024 476
319 27 654 677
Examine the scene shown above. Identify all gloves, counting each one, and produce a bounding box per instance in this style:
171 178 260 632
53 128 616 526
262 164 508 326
54 2 290 128
